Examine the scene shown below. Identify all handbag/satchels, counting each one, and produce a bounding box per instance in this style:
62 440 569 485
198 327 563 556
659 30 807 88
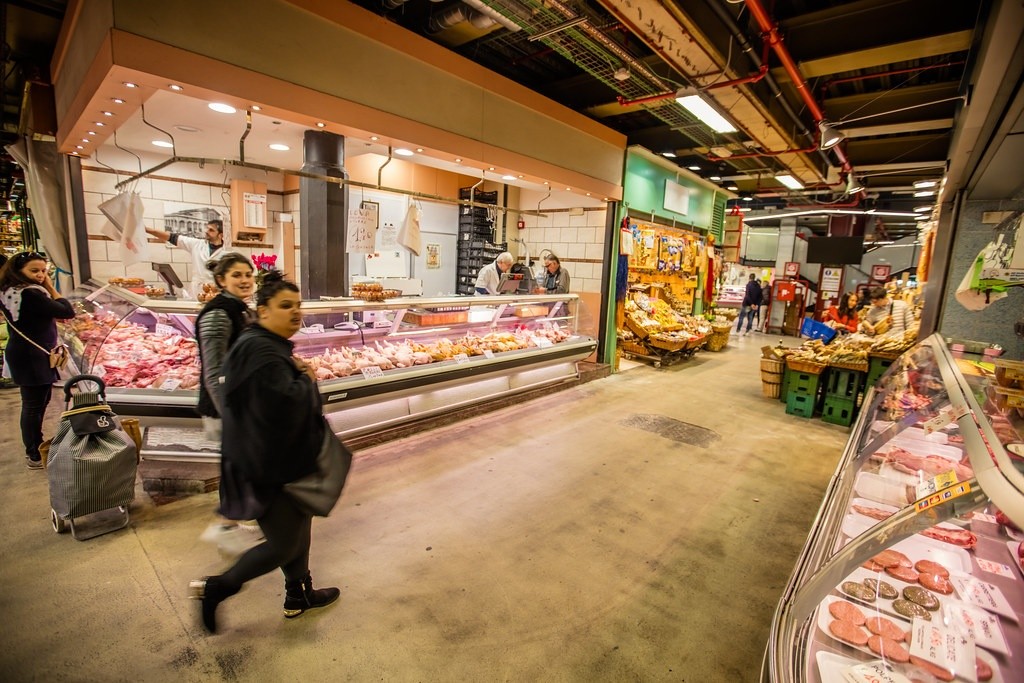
50 345 69 371
281 415 353 517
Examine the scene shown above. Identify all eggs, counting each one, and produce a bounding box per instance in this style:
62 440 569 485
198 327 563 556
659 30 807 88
352 281 398 302
197 282 222 301
108 277 144 285
146 287 165 297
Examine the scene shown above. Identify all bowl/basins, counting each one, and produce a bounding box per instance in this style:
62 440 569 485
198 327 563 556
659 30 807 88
987 359 1024 418
984 348 1003 356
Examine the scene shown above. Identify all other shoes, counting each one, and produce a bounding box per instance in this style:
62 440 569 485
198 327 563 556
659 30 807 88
25 454 44 469
201 522 268 551
743 332 749 336
755 329 762 332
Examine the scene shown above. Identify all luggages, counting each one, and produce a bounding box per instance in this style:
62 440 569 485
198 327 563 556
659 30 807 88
46 374 137 540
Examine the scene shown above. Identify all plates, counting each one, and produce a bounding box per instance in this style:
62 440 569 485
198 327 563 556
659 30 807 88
1007 444 1024 457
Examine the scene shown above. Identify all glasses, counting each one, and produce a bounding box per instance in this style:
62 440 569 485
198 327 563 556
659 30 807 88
545 263 551 267
20 251 47 258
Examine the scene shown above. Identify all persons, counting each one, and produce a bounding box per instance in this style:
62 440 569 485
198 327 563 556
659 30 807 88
821 284 916 338
143 219 340 637
532 255 570 325
735 274 771 336
475 252 514 295
0 252 76 469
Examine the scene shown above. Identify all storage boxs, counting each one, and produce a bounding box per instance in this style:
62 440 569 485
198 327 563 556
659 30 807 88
779 357 893 427
455 186 507 296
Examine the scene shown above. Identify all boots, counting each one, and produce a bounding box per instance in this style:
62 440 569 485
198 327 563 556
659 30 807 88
189 572 228 633
284 570 340 618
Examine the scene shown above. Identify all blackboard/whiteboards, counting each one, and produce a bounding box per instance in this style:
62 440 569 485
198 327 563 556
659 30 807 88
663 178 689 218
344 186 460 296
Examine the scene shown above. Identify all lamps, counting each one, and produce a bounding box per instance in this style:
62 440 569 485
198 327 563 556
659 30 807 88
774 169 806 190
674 85 739 134
818 119 845 151
845 173 865 196
912 177 942 221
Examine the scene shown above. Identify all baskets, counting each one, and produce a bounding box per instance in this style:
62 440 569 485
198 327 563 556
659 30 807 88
650 335 706 351
623 314 649 355
786 355 827 375
827 361 868 372
705 324 732 352
761 358 784 399
868 352 899 359
801 311 837 344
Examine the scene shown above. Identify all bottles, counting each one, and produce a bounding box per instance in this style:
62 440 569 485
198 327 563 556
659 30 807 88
369 314 377 322
